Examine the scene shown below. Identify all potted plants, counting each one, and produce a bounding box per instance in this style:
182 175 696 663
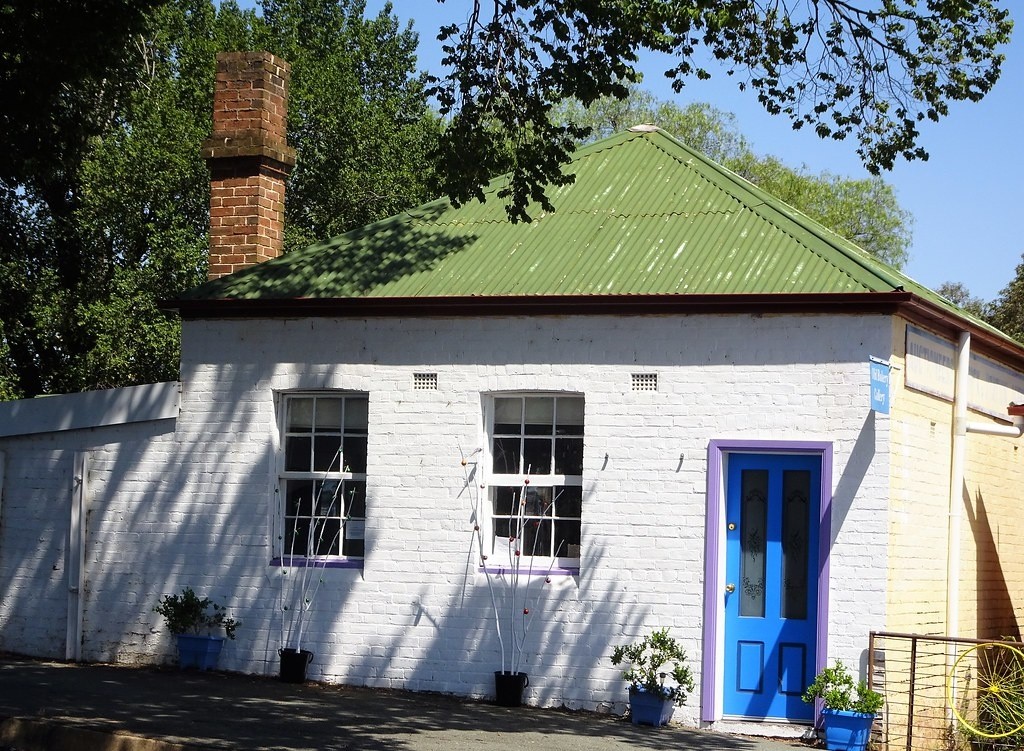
610 627 696 727
152 586 242 671
801 657 886 751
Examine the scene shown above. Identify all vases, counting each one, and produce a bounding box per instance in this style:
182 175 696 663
493 671 529 706
278 648 314 684
